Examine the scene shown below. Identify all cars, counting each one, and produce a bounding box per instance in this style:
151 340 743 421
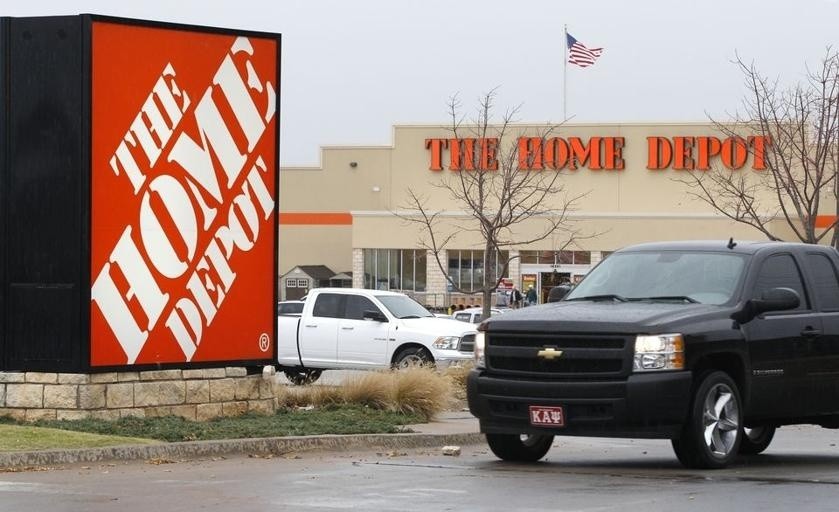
278 297 512 326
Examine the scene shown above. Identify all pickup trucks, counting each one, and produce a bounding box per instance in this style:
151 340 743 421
277 285 479 388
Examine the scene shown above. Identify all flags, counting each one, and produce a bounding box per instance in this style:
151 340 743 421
565 32 604 71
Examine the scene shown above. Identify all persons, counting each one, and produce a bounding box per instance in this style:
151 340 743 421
510 285 523 309
525 284 537 306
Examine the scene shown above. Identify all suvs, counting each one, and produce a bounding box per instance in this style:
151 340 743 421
464 237 838 470
